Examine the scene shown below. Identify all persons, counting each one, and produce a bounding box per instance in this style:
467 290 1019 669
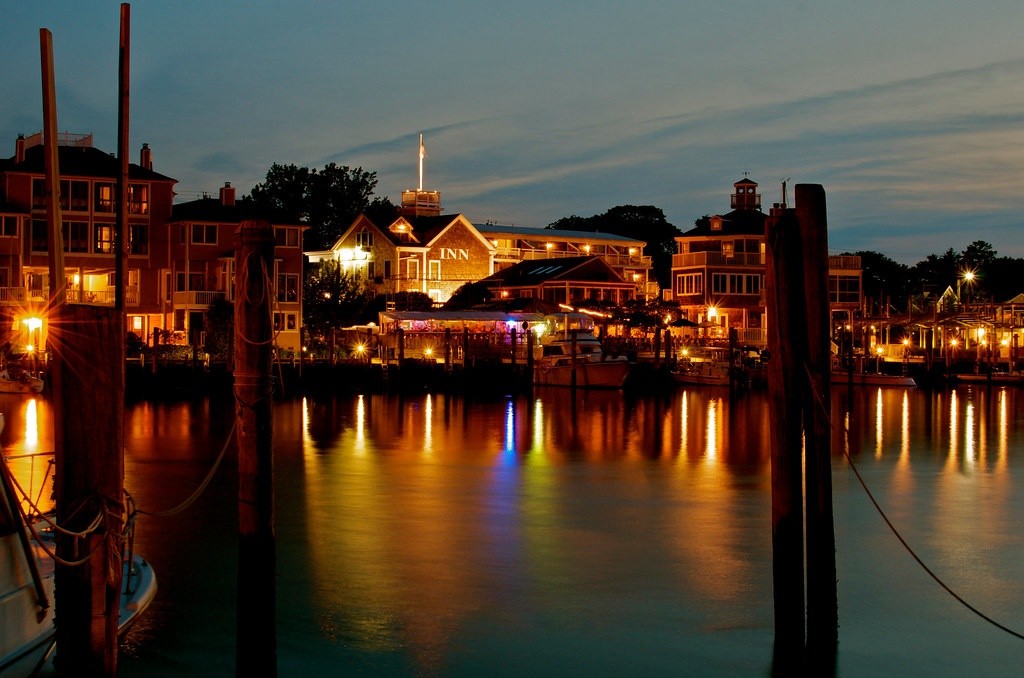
672 335 691 347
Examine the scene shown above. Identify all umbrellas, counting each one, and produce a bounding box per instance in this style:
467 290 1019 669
668 318 698 348
691 320 721 337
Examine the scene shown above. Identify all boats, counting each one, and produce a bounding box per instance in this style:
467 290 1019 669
534 312 630 389
661 351 731 386
